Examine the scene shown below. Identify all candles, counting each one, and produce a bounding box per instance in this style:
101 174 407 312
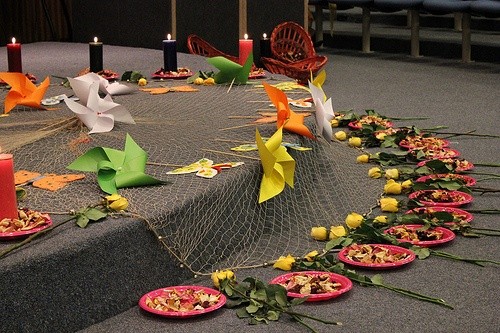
163 34 177 73
261 34 272 57
239 33 253 67
7 36 22 73
0 153 18 223
89 36 103 73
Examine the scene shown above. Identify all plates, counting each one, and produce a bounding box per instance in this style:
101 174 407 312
416 174 477 187
405 206 474 225
270 271 353 301
248 72 266 78
338 244 416 270
0 212 52 240
418 160 474 172
348 120 424 136
138 286 227 317
384 225 456 248
399 141 448 147
409 189 473 208
151 70 194 79
78 70 120 81
410 148 460 159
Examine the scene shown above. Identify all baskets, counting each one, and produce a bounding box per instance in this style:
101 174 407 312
262 21 327 86
188 35 239 63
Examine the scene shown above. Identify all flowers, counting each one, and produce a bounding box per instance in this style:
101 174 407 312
309 225 500 268
121 70 148 86
186 69 216 86
330 109 500 195
209 269 340 333
269 251 455 311
0 194 129 258
379 197 500 215
344 211 500 237
